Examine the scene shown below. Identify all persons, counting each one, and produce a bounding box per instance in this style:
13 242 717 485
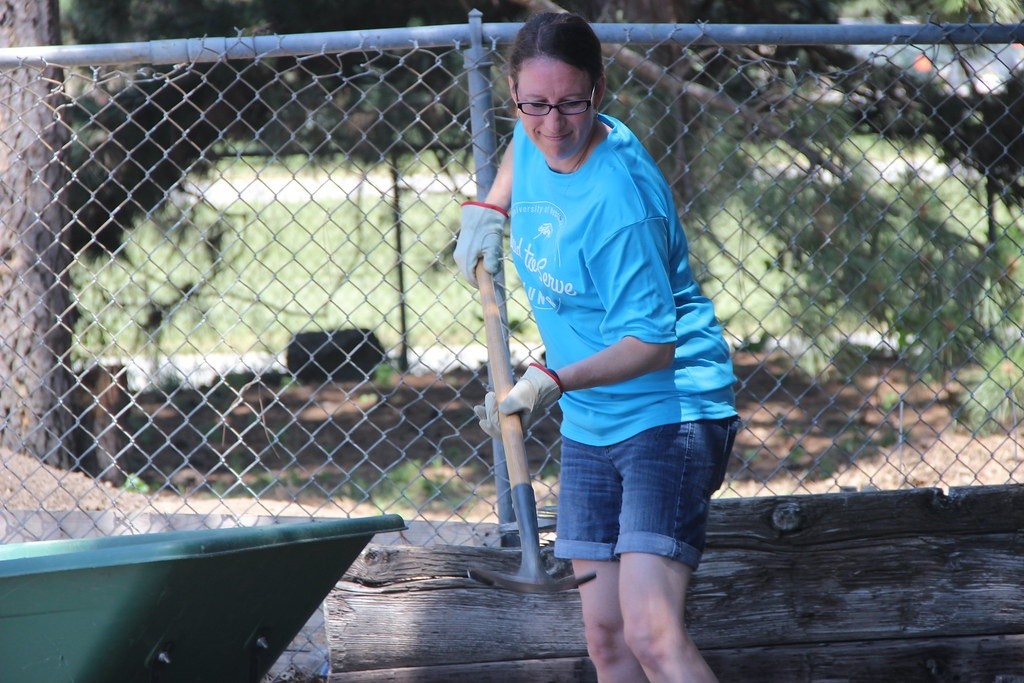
454 11 740 683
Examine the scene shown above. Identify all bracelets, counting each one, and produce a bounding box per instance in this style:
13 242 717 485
546 368 559 379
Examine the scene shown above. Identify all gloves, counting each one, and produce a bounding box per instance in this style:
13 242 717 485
473 362 565 441
453 201 510 291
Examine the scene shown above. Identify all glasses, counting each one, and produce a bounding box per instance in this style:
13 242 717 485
514 82 598 116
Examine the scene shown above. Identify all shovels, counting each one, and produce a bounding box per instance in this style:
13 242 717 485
466 256 598 593
